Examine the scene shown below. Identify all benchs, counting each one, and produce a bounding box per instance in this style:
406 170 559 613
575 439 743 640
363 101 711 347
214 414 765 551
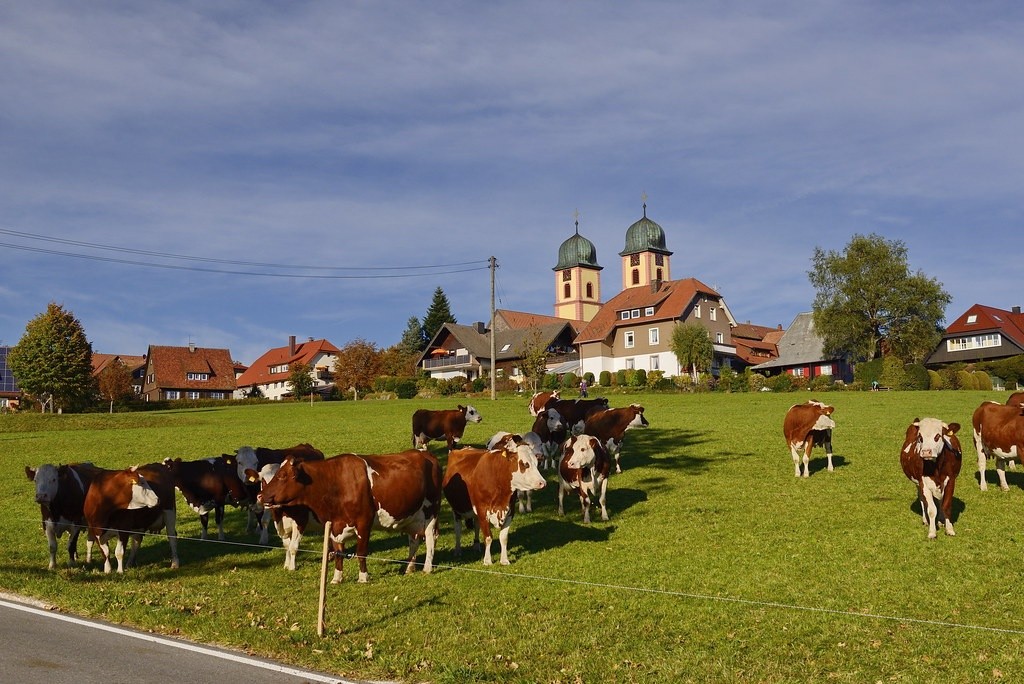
872 386 893 391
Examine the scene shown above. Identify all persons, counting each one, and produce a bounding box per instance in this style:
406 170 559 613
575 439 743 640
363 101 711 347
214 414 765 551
578 379 588 398
871 382 880 391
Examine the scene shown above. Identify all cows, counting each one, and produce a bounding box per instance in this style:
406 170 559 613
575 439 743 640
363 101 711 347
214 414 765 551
783 398 836 479
899 417 962 538
971 391 1024 492
24 387 650 584
